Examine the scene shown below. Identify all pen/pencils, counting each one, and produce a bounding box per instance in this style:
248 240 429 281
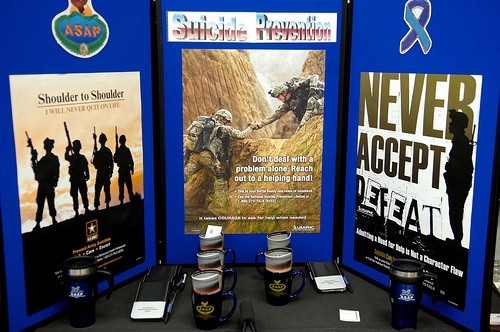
341 271 353 294
180 273 187 291
164 290 177 324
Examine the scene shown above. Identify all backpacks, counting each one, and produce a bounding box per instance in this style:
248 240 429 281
286 76 324 88
185 115 221 152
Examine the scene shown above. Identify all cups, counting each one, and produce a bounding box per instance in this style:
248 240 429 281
389 259 439 331
256 230 292 277
196 248 237 289
191 268 237 330
199 231 235 268
255 247 307 307
63 256 112 328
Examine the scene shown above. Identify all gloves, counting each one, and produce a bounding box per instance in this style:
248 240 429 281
307 90 319 100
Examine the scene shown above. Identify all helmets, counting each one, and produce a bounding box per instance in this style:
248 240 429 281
216 109 233 124
272 83 289 97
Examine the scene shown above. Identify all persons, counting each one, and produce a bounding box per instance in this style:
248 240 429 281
250 74 324 134
183 108 257 205
